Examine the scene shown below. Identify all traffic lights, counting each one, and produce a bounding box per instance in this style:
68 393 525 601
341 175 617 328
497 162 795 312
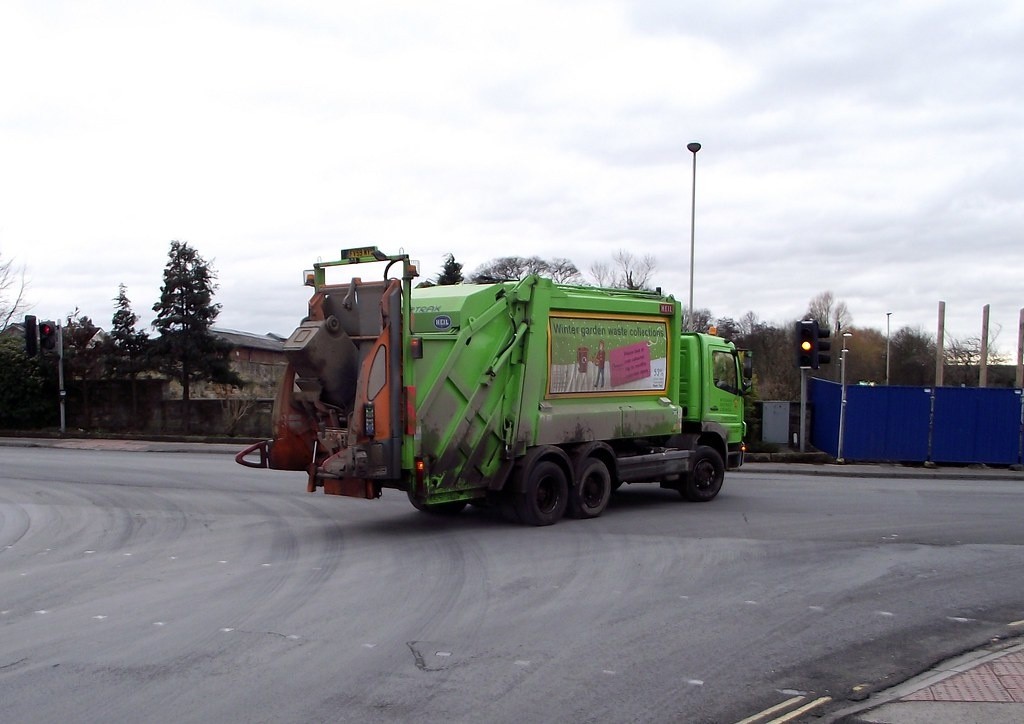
39 321 56 350
794 320 817 370
816 328 831 365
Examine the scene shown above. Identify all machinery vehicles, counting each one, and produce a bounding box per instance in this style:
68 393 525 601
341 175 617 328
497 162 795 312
235 245 755 527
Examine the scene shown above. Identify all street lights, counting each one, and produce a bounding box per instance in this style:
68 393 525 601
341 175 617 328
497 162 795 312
840 331 853 459
886 312 892 384
